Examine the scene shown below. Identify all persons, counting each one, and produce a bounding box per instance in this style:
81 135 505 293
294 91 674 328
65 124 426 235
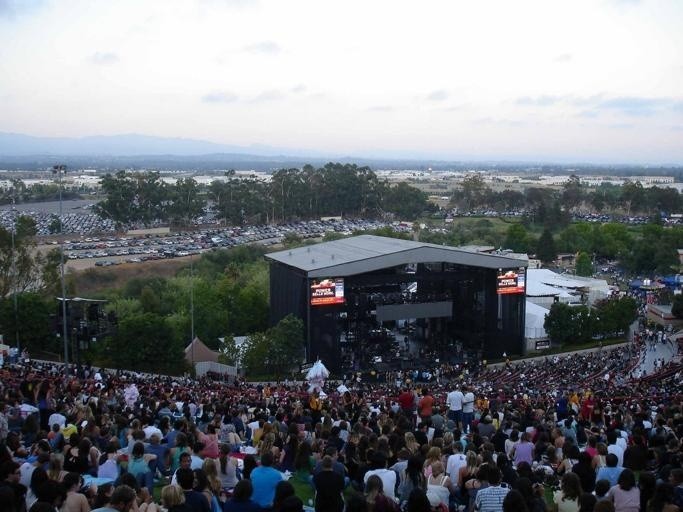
1 326 683 510
